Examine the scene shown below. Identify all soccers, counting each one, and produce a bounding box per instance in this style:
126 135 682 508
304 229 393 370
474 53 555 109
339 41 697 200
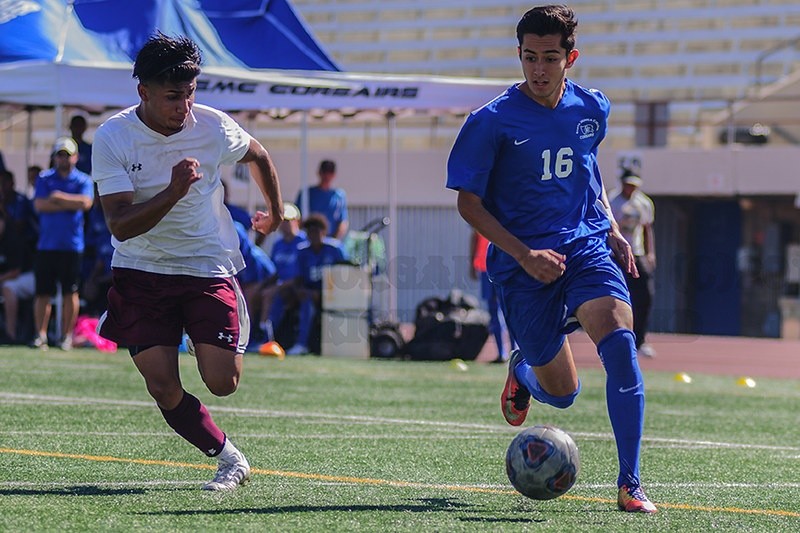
505 425 579 500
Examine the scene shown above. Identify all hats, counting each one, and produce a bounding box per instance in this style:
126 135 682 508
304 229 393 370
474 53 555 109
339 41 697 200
621 169 642 187
282 203 301 222
53 137 77 158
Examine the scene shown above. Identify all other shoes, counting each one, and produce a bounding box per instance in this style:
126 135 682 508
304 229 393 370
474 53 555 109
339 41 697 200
287 344 307 355
29 335 47 348
636 344 657 358
59 338 75 352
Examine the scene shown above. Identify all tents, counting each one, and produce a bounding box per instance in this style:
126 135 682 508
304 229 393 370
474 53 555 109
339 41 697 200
1 1 513 321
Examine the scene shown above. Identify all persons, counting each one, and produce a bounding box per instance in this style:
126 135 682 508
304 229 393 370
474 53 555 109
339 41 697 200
607 155 654 357
92 29 286 491
446 3 658 514
467 229 519 365
0 112 350 356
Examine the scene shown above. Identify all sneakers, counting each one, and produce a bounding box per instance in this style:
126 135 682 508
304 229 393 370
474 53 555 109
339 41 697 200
185 334 196 356
203 452 251 490
501 349 532 427
617 484 657 513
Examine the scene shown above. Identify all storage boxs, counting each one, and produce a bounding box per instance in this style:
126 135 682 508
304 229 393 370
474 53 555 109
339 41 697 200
318 264 369 358
